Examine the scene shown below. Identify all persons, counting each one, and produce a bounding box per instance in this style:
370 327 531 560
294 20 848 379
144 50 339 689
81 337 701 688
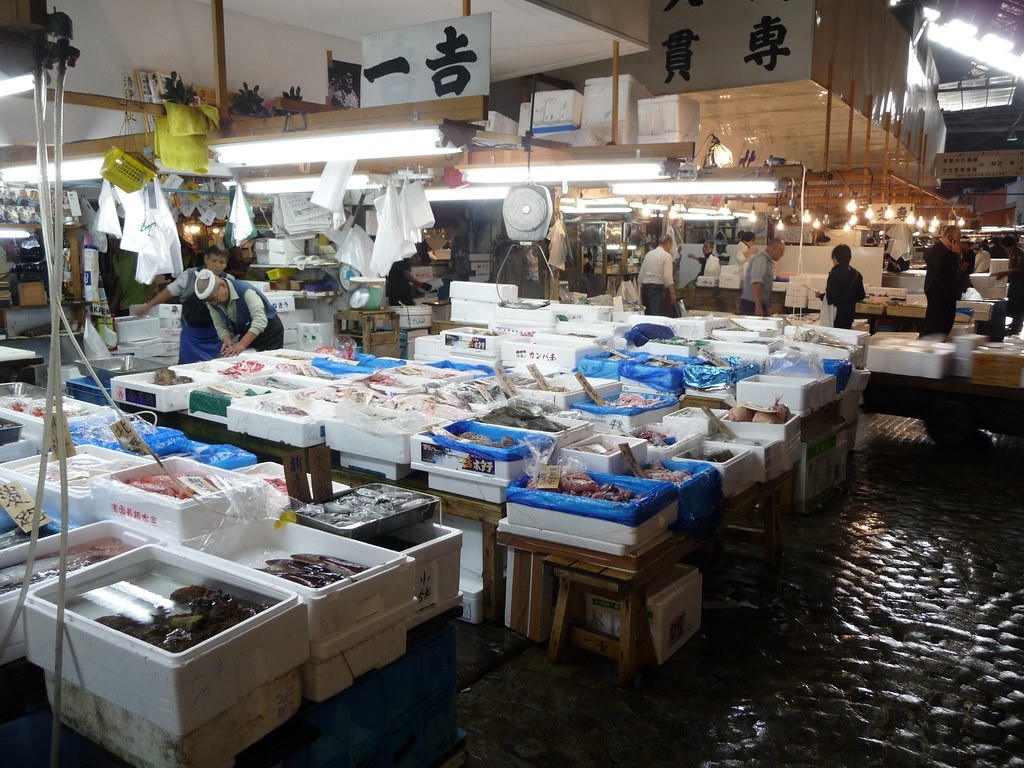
194 269 284 358
992 236 1024 337
110 240 156 316
583 246 615 273
443 236 466 273
637 234 676 317
135 244 235 365
738 238 786 316
815 243 866 329
959 235 1010 287
171 235 246 279
2 190 39 207
919 225 968 343
412 240 437 265
688 241 715 274
864 237 927 272
388 259 432 305
729 231 758 266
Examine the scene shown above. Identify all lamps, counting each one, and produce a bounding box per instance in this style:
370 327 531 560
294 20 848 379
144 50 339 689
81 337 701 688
679 133 733 175
0 71 788 223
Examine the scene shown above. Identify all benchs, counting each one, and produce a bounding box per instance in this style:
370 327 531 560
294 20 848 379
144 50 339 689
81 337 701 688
548 468 791 685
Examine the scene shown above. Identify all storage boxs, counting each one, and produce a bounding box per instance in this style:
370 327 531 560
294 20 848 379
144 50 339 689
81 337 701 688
66 375 112 406
471 103 573 151
636 96 700 143
969 259 1009 300
246 280 335 350
581 74 655 146
785 276 927 317
99 150 157 194
531 89 585 132
697 266 787 293
46 366 87 389
1 281 872 768
469 254 492 282
864 332 1024 389
112 303 182 364
386 305 431 329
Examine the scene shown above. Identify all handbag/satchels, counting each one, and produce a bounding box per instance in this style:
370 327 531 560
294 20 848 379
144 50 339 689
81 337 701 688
819 294 837 328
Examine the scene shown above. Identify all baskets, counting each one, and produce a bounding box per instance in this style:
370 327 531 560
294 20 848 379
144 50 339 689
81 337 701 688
100 148 155 194
265 268 294 279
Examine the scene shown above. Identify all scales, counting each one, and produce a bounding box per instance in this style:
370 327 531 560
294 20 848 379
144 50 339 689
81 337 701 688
350 277 386 310
496 184 554 310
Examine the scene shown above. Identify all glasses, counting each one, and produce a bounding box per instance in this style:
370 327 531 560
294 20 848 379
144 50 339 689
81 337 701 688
203 286 220 306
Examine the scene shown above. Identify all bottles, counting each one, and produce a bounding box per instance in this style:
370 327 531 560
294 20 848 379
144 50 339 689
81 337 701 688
0 186 67 224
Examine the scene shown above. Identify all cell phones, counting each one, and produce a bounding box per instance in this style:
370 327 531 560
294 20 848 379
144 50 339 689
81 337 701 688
947 235 956 244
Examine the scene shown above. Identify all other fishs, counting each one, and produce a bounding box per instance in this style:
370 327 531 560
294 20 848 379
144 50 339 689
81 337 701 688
0 553 76 586
257 553 370 590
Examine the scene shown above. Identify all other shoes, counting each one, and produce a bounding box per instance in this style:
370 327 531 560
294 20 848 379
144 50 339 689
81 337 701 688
1004 328 1010 331
1004 330 1014 336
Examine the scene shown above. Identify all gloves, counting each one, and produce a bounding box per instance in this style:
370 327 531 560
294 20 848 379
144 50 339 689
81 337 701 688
421 282 433 292
